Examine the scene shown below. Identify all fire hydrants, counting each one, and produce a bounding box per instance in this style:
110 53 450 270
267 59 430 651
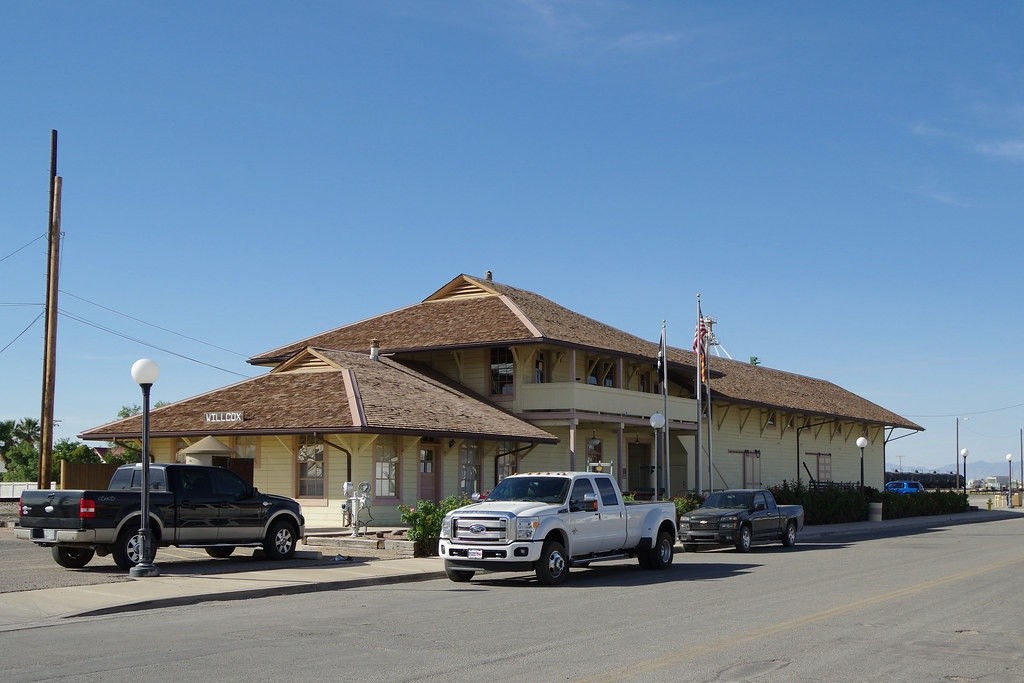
986 497 993 512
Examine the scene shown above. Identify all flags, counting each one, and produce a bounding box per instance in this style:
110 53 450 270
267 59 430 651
692 307 709 385
656 329 664 383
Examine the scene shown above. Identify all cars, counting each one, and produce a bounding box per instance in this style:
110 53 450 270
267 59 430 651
884 480 925 496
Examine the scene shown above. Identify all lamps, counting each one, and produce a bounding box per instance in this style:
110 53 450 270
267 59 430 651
755 450 760 458
635 432 640 445
745 449 749 456
590 429 601 446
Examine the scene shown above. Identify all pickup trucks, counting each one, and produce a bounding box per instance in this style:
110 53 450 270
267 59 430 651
438 471 684 586
679 488 805 553
14 462 305 572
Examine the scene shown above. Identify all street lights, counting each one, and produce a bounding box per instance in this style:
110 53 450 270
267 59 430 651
1006 453 1014 508
856 437 868 495
130 358 160 577
961 448 969 494
650 411 665 501
